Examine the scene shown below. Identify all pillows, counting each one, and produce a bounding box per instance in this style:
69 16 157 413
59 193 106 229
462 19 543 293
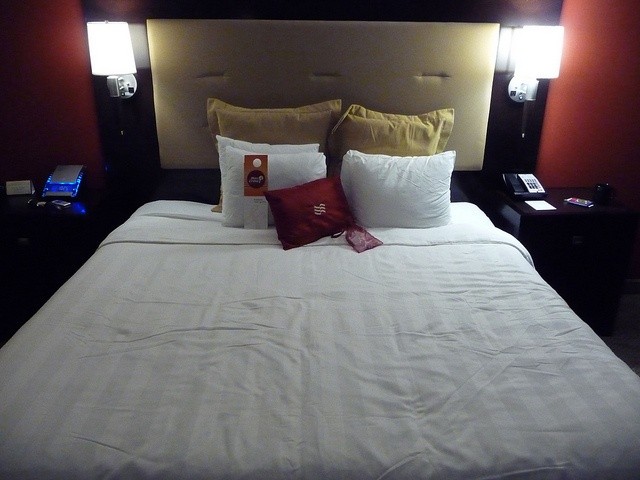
263 173 357 256
215 145 327 227
205 95 341 155
329 102 458 155
213 133 321 184
339 150 456 228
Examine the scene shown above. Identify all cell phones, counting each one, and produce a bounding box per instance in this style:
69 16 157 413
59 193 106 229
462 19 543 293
564 197 595 209
51 199 71 209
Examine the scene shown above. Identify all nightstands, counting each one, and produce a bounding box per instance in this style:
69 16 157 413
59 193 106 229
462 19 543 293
501 187 636 337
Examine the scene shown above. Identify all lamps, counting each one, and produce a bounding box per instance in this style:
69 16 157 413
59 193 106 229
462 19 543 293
85 21 140 136
508 24 565 140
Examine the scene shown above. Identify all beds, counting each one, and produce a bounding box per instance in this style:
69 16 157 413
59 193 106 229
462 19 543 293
1 199 640 480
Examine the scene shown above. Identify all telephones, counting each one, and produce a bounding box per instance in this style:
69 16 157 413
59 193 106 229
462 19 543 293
502 173 546 200
